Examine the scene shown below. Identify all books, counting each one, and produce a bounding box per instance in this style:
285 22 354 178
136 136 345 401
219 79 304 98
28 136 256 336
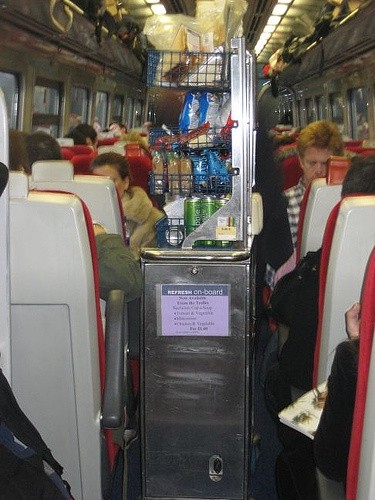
277 379 327 440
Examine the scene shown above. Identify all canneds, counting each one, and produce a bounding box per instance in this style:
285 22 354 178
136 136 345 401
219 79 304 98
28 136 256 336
165 195 235 248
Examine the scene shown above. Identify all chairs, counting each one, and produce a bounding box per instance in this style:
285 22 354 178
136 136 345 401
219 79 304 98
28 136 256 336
0 127 375 500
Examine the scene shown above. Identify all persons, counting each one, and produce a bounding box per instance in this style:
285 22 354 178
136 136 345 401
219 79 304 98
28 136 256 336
7 129 145 305
310 298 363 483
276 115 292 132
270 153 375 391
88 152 165 248
26 129 63 172
64 124 98 151
259 119 346 294
108 122 128 139
